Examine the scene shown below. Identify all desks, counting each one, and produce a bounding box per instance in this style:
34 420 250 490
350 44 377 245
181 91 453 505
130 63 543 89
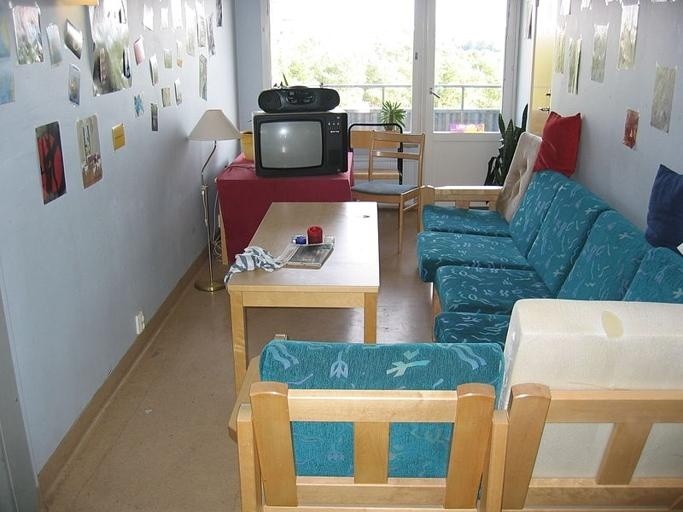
216 151 353 265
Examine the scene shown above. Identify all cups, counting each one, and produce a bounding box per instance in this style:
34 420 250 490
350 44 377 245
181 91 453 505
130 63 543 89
307 225 323 243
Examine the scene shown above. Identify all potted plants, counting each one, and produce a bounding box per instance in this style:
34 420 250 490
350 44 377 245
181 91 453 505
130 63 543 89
380 100 407 131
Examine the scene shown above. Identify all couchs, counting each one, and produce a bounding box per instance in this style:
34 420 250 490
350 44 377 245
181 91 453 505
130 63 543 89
415 166 683 342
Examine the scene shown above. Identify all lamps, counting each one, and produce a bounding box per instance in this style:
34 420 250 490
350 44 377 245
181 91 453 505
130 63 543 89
187 110 244 292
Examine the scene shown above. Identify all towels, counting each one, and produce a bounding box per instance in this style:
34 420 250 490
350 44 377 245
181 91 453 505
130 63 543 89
223 244 287 287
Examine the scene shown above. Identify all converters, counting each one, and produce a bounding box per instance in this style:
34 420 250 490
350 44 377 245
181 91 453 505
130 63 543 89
218 215 220 227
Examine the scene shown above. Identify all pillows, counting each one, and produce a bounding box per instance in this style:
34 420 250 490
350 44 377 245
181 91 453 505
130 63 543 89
644 163 682 249
532 109 583 179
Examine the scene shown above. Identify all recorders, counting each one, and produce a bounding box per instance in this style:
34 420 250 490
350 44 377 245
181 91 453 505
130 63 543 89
258 86 340 112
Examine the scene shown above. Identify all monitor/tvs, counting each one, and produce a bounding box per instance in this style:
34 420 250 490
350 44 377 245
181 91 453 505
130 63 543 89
252 111 348 178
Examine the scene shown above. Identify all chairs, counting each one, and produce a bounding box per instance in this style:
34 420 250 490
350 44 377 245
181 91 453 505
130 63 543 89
227 331 506 512
478 383 683 512
350 130 426 255
348 122 404 185
